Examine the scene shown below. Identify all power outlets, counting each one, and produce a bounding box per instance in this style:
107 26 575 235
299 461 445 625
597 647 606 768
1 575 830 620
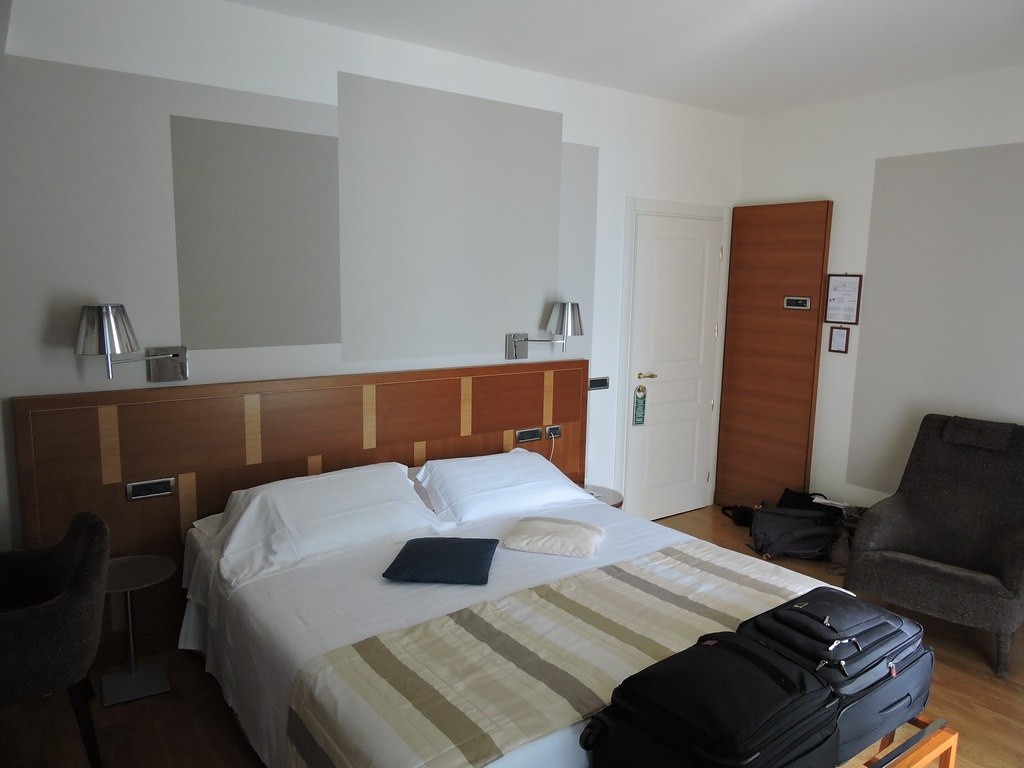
545 425 561 440
515 428 542 443
126 478 176 501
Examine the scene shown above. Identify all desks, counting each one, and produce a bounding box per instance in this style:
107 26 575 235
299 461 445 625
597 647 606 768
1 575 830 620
101 555 176 707
838 716 959 768
578 483 623 508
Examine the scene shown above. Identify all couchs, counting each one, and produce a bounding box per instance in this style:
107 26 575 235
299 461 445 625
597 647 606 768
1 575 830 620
842 414 1024 679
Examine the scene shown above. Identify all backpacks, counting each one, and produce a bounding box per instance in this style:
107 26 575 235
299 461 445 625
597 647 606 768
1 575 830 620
720 488 851 561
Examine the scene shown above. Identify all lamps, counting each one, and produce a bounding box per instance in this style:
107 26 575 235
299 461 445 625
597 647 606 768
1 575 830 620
73 303 189 382
505 301 583 361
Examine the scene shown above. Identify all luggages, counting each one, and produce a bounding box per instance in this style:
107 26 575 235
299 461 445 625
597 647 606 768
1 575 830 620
578 587 934 768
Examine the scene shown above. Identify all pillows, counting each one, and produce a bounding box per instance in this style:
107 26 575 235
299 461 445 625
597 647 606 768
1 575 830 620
221 462 456 583
416 452 602 527
382 537 499 586
503 517 606 556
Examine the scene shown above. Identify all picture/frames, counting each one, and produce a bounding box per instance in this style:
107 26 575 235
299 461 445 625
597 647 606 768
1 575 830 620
828 325 850 354
824 272 863 325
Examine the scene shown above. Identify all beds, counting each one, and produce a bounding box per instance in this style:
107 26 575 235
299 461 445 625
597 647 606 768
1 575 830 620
177 468 856 768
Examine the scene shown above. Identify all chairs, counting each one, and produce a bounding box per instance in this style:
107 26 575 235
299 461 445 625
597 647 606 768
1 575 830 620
0 514 109 768
